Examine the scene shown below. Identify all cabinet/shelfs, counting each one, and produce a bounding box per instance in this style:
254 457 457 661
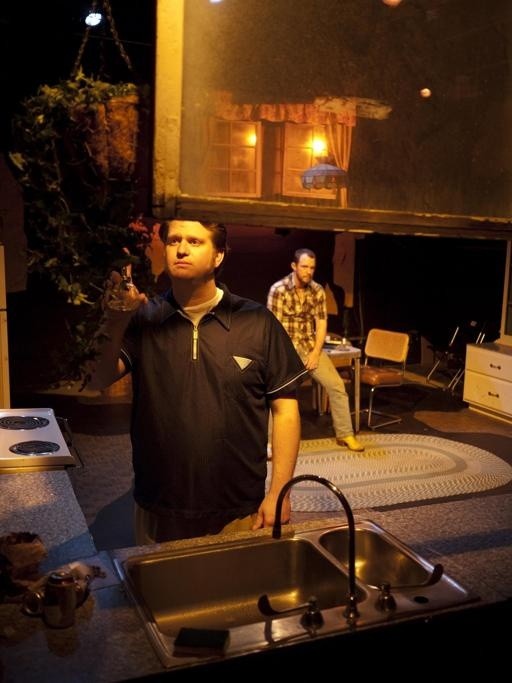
462 342 512 423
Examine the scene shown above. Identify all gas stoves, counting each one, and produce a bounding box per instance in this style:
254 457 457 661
0 405 78 474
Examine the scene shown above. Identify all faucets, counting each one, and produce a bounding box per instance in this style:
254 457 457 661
272 476 369 618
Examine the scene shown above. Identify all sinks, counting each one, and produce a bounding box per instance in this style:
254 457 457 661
304 516 485 621
118 534 378 668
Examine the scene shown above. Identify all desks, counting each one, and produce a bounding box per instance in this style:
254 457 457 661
311 333 362 431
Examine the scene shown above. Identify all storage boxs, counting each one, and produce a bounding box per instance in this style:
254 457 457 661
270 122 342 203
182 112 264 199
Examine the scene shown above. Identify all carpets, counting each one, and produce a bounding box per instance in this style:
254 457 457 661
264 434 512 512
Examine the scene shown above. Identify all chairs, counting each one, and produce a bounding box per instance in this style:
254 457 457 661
350 330 409 429
426 322 486 396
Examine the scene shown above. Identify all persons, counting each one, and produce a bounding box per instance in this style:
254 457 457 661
265 248 364 453
90 220 307 541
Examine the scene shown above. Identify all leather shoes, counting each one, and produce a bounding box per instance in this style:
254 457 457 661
337 436 365 452
